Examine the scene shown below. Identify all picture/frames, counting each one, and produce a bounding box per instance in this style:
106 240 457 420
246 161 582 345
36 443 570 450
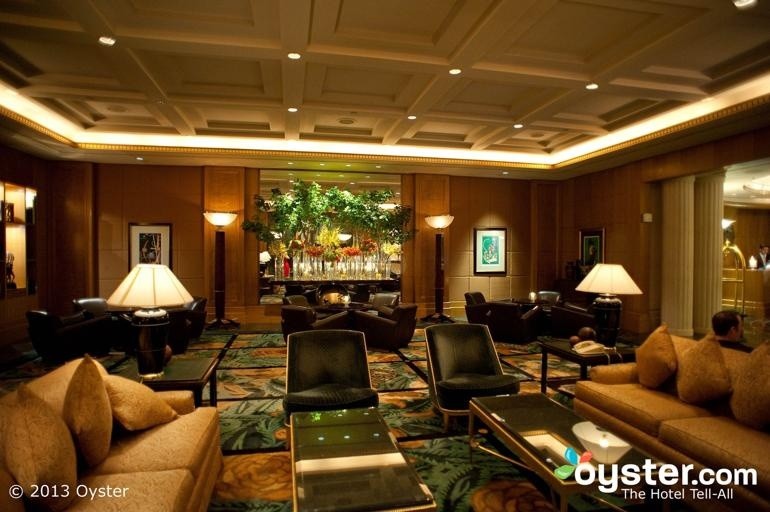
472 226 508 276
126 222 174 278
578 225 606 272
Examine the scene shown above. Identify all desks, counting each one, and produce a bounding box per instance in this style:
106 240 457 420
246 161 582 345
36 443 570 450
536 338 637 402
104 355 221 411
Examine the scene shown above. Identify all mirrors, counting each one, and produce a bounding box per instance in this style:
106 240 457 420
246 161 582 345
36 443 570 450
253 165 405 305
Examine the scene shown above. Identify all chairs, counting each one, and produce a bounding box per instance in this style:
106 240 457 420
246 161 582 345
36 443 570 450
255 278 422 353
421 322 520 438
23 293 211 369
281 329 380 452
462 284 611 345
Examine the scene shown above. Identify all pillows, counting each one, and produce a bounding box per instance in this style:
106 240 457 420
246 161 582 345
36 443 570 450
100 373 180 435
1 380 82 510
61 355 114 471
725 339 770 431
674 331 735 404
633 322 678 392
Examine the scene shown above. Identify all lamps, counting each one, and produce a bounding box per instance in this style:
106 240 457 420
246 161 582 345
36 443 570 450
574 261 645 348
424 214 455 231
200 207 239 228
102 263 195 378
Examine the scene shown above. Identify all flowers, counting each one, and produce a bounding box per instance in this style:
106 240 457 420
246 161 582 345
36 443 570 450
265 237 379 280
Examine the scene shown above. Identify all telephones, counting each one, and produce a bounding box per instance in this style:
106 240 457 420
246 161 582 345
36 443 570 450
571 339 605 356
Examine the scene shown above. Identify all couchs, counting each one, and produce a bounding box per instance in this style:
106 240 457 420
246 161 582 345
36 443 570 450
569 333 769 512
1 354 226 512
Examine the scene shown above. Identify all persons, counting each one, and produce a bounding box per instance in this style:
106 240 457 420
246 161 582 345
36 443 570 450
707 309 753 354
270 283 281 294
748 242 769 268
